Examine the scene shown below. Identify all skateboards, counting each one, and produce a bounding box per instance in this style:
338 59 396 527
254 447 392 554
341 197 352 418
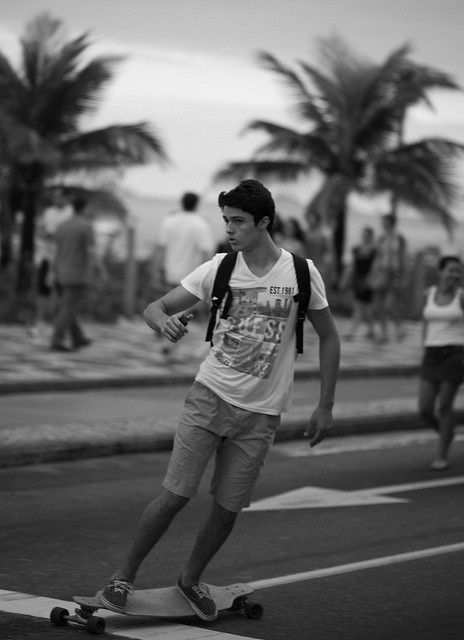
50 582 263 634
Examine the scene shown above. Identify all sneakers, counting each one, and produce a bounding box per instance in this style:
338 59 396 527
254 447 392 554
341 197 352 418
99 575 132 613
176 576 218 622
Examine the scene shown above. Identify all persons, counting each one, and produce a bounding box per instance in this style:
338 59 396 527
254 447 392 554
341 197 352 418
156 192 214 288
282 219 303 257
304 210 326 262
49 197 93 350
343 227 376 341
378 210 405 340
29 259 50 338
97 179 340 621
418 256 463 467
